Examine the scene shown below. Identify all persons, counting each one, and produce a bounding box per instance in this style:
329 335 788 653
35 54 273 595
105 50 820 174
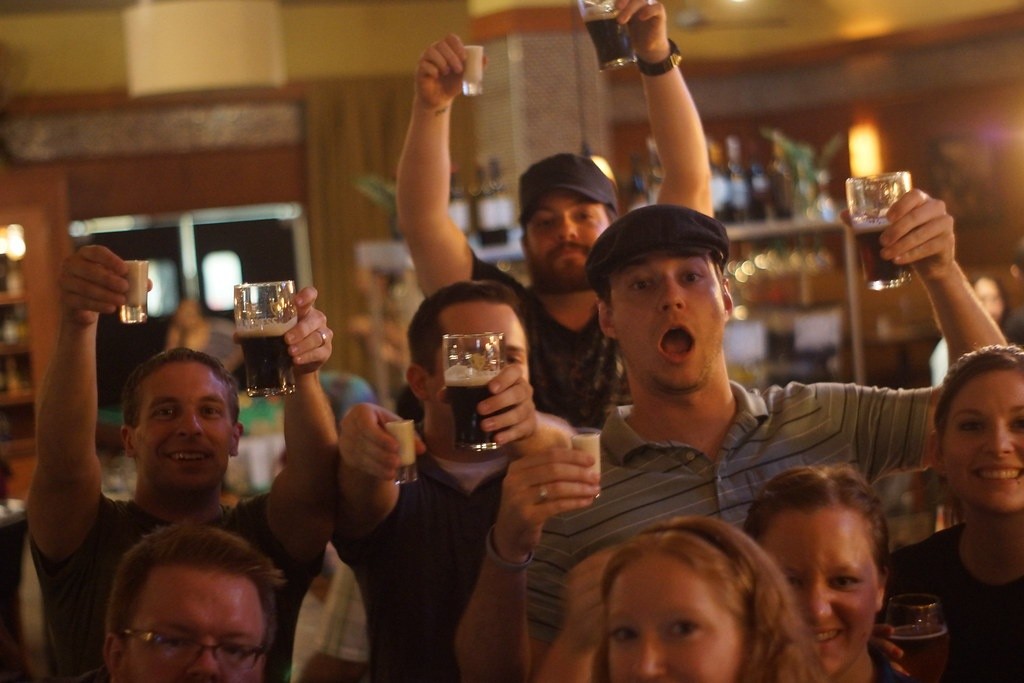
397 0 711 433
600 515 829 683
163 298 210 352
330 281 577 683
929 273 1003 386
103 522 277 683
27 245 339 683
538 466 912 683
456 190 1009 683
867 346 1024 683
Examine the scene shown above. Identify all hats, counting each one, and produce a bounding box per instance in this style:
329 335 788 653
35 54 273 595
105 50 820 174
585 206 728 296
517 153 618 213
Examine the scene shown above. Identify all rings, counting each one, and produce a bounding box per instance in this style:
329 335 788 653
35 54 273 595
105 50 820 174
537 484 548 503
315 331 327 347
646 0 654 6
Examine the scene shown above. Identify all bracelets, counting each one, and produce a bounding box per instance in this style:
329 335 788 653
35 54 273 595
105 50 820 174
486 524 535 572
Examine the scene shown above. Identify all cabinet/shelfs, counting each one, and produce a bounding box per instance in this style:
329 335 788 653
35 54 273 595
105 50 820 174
727 216 865 389
0 224 36 455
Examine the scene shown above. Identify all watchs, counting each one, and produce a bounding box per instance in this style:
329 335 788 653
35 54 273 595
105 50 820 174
636 38 681 77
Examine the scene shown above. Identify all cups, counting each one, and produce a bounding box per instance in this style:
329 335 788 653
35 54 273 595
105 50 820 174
440 332 501 451
231 280 298 399
572 0 637 71
115 261 148 325
570 430 602 501
881 594 949 683
458 46 486 100
843 174 916 289
384 419 419 484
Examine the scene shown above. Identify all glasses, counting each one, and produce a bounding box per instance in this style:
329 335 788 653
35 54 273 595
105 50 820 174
119 628 264 673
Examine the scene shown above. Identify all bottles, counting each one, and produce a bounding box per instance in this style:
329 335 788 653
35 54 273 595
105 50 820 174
626 128 840 304
